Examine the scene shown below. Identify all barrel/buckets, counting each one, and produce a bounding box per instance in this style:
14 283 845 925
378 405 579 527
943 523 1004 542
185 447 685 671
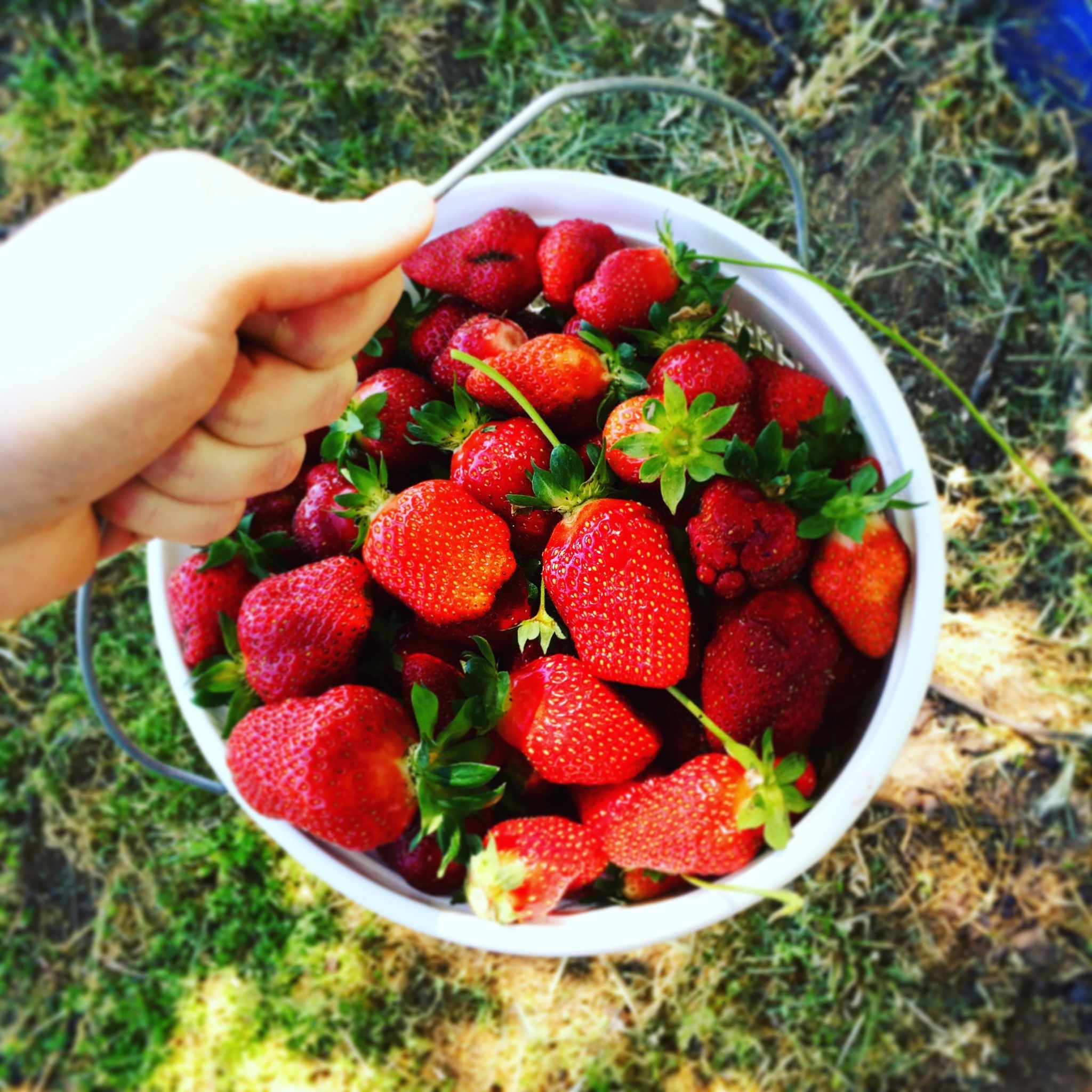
75 74 950 959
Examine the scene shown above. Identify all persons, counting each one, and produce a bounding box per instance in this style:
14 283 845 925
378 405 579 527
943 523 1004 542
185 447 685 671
0 148 435 622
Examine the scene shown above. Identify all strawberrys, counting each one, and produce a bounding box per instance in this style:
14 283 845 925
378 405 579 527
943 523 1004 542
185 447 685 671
169 205 928 928
